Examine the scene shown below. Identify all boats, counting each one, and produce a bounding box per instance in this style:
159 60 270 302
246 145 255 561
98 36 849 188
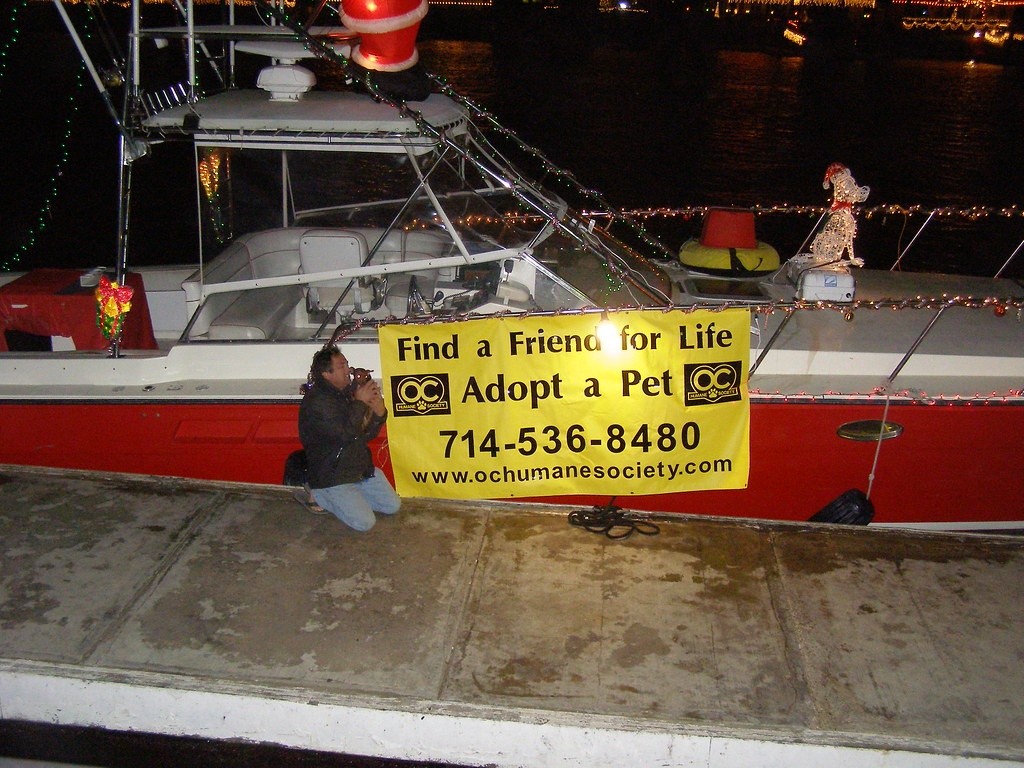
1 0 1024 531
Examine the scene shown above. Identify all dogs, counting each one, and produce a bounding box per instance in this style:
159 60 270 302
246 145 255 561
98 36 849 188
788 164 870 275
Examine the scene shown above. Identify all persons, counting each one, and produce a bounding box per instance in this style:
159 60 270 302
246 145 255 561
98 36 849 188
293 341 402 531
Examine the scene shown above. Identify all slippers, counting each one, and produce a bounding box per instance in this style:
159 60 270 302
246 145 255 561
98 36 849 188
293 482 330 515
